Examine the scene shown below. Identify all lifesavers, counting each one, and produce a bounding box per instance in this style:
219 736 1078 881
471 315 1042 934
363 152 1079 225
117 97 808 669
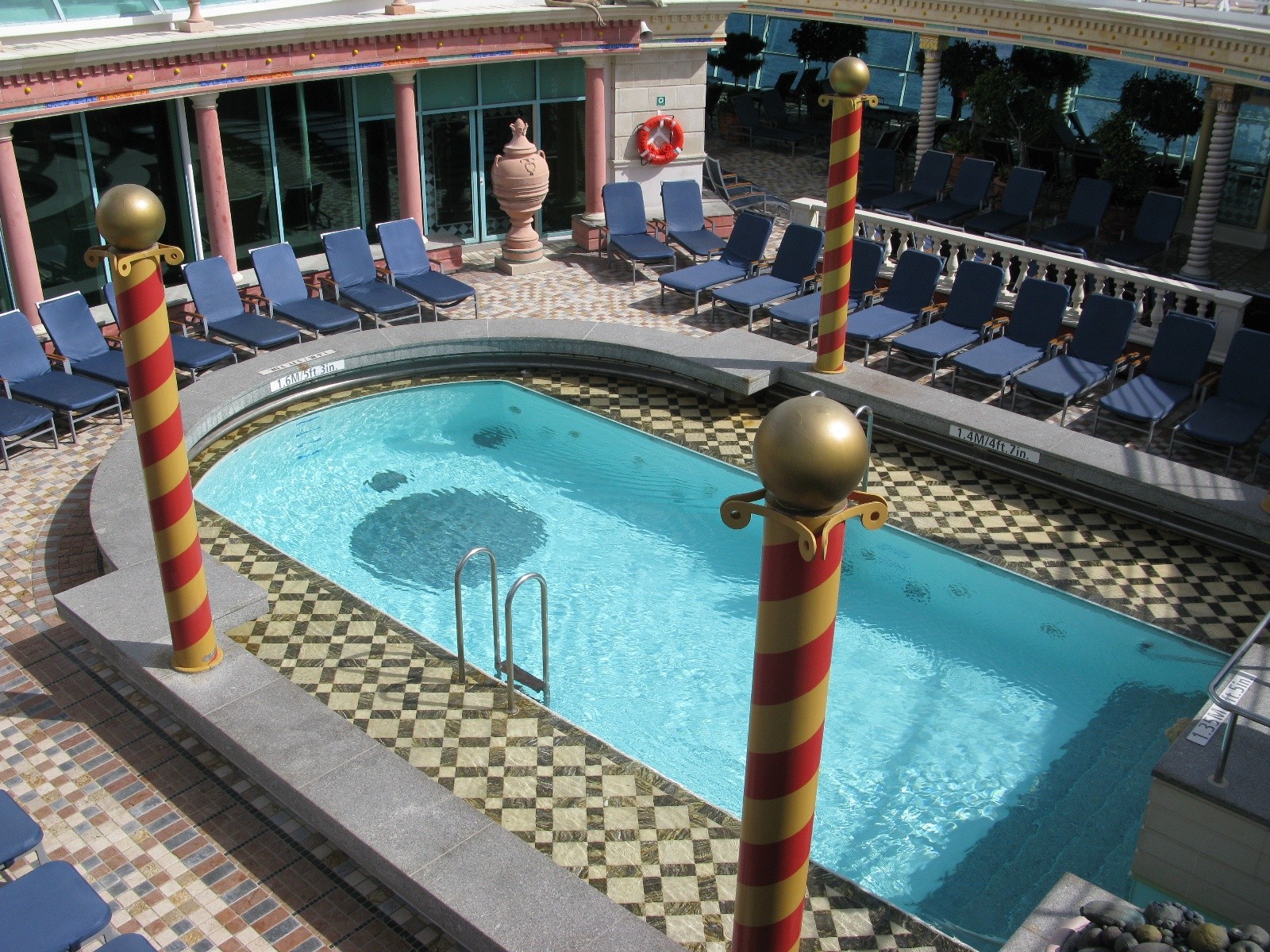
636 116 683 167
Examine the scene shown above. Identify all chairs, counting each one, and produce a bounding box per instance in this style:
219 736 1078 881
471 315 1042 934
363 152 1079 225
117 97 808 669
884 260 1010 388
657 210 776 315
176 255 302 357
248 241 363 341
768 234 888 349
653 180 728 267
1091 310 1220 453
316 226 422 330
0 377 59 470
1 788 45 870
949 276 1073 409
0 858 111 952
845 249 947 369
708 64 1188 274
0 309 124 444
34 291 135 419
1012 294 1141 428
596 181 676 286
1168 327 1270 478
103 281 238 383
48 183 334 288
375 216 478 322
710 222 825 332
